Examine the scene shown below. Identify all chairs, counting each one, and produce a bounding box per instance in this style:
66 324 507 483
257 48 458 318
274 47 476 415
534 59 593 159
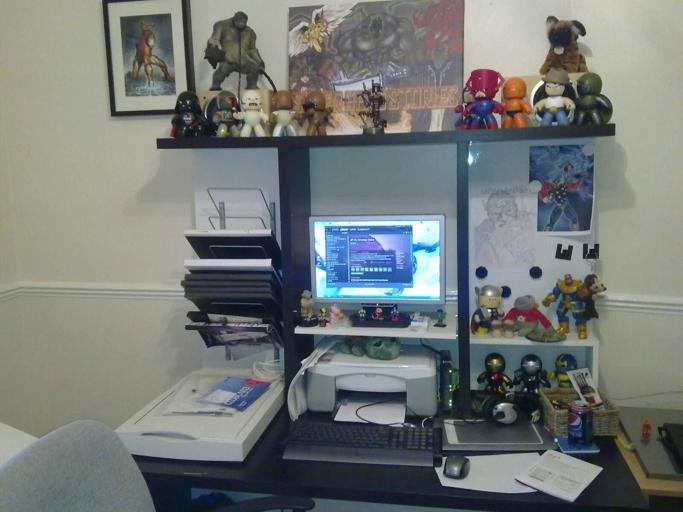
0 418 315 512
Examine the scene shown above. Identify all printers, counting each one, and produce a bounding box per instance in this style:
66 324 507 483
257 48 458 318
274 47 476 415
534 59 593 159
306 344 437 425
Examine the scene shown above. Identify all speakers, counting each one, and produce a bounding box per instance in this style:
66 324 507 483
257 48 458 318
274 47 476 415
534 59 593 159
196 90 274 139
501 73 590 129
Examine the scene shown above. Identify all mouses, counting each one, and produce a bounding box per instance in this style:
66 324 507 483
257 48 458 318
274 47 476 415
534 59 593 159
444 453 469 478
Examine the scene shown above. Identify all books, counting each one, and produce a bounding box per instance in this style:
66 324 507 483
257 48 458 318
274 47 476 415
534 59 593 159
180 189 281 321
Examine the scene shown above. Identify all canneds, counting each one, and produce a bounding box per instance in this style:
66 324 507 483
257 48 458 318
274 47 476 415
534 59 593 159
568 400 593 449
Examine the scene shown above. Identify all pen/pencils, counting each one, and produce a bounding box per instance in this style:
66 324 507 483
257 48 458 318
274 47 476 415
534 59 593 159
545 426 559 443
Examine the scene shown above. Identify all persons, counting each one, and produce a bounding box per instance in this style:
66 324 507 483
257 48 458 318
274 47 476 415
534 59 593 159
472 274 604 414
453 16 613 129
171 88 333 136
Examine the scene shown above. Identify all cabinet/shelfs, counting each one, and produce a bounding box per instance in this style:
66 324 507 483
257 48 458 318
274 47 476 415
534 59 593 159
155 124 615 419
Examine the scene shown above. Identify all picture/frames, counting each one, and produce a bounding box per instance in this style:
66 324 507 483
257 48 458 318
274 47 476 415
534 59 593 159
102 0 196 116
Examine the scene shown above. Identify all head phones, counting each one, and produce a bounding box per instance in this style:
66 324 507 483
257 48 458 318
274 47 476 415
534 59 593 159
484 392 542 425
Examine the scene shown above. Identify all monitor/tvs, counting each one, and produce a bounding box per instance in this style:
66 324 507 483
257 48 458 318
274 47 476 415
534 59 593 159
308 214 446 328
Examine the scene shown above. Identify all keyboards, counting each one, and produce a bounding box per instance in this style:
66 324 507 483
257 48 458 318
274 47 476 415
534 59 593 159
282 422 442 467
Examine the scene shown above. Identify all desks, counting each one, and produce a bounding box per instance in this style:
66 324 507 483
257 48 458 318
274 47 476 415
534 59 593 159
132 394 650 512
616 433 683 505
